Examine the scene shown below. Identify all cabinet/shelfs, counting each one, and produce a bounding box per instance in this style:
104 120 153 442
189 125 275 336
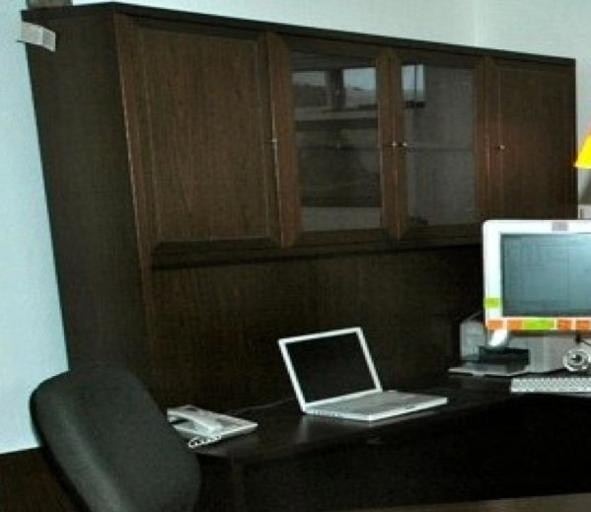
20 2 580 418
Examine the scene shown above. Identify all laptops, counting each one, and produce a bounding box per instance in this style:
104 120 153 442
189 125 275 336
278 326 448 420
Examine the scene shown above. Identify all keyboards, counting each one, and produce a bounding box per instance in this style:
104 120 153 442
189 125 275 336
510 377 590 394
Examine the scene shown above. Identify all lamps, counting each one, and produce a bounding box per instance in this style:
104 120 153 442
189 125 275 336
571 129 591 220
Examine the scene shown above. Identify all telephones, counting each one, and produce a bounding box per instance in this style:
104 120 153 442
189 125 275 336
166 404 258 444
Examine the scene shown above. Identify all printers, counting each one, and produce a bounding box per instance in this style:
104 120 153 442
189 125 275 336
448 312 576 378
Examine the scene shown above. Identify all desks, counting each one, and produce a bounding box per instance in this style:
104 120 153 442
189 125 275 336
197 363 591 512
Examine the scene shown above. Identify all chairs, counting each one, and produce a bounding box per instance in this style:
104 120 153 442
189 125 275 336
30 362 203 512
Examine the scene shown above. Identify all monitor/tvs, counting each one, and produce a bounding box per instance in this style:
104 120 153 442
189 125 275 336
481 217 591 334
